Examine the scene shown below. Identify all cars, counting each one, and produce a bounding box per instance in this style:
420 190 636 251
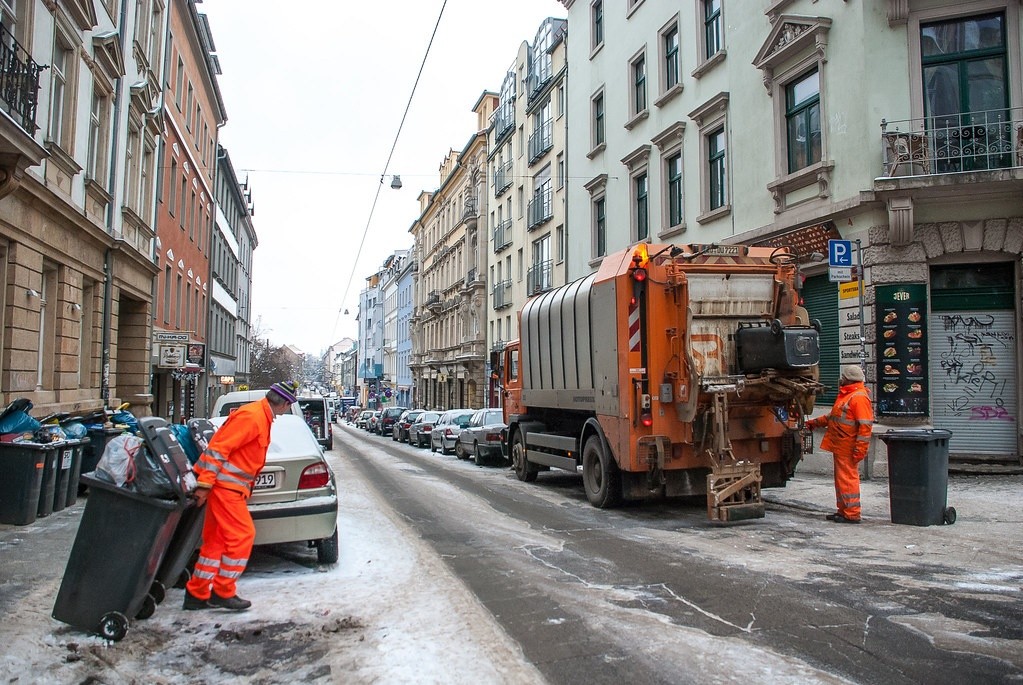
304 381 361 423
454 407 509 465
407 409 446 449
354 410 375 429
392 408 428 444
365 411 383 432
207 413 339 564
430 407 483 454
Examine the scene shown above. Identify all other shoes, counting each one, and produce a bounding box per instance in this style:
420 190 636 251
206 587 251 609
181 588 217 610
826 513 860 524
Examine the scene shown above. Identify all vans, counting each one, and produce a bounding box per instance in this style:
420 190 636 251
210 390 304 421
295 395 332 450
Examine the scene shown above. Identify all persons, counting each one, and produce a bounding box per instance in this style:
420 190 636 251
802 365 876 522
178 379 297 607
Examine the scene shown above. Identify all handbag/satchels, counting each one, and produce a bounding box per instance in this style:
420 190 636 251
0 408 202 502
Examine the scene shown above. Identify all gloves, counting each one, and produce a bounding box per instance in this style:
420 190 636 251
804 419 816 432
852 449 864 465
192 488 210 508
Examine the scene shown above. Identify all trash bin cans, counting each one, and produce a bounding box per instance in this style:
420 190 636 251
734 318 823 376
148 417 219 608
878 427 957 527
0 402 139 525
50 416 199 642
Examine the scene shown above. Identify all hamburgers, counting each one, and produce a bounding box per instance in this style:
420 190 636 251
883 346 897 358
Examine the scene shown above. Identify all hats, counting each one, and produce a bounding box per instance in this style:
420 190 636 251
841 365 865 382
269 380 299 404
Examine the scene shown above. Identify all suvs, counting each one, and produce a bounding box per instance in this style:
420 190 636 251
375 406 408 437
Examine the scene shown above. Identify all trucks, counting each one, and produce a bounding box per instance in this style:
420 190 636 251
489 242 833 523
